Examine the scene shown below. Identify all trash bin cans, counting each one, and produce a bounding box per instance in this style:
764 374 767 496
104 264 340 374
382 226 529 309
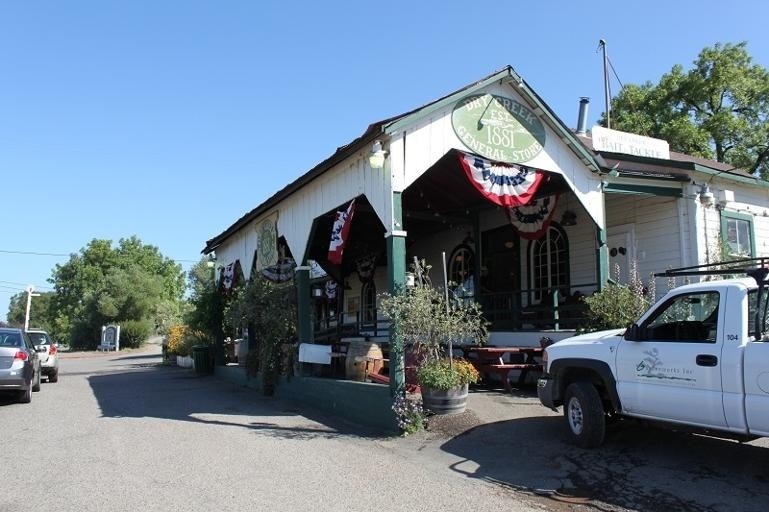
192 345 208 373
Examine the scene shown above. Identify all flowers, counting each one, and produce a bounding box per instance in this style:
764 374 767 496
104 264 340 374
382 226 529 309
413 354 483 391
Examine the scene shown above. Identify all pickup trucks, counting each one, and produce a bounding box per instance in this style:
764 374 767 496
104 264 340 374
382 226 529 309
537 256 769 447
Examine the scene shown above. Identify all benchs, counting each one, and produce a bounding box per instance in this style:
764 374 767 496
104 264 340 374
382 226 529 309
448 341 547 396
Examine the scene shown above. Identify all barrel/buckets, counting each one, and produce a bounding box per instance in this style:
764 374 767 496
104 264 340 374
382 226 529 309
345 341 385 383
419 387 468 414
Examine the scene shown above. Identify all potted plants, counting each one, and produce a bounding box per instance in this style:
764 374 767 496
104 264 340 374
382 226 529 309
376 252 493 412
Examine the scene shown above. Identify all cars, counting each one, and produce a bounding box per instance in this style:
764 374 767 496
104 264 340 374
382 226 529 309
26 329 59 382
0 327 41 402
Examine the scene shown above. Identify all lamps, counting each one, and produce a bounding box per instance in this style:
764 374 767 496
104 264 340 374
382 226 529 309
368 139 390 169
560 191 578 226
503 77 524 88
691 179 715 210
206 253 218 268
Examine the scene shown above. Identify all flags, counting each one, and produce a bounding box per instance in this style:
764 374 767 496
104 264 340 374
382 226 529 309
327 200 355 265
